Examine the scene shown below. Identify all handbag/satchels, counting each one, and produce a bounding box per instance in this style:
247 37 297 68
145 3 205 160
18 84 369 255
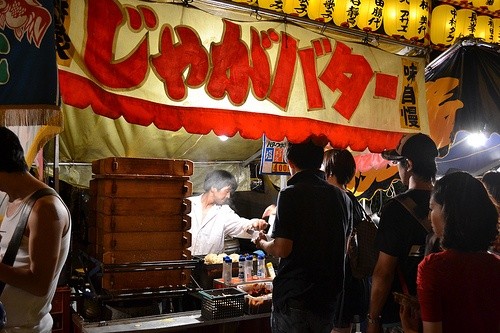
348 190 379 255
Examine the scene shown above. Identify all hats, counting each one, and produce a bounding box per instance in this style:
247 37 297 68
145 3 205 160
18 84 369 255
382 133 437 160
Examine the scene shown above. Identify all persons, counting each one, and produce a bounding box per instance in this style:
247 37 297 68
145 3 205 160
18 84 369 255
0 123 71 333
482 170 500 257
250 136 353 333
319 148 370 333
367 133 438 333
417 167 500 333
186 170 268 257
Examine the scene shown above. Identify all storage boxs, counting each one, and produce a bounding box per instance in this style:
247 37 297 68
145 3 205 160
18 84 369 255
199 281 273 321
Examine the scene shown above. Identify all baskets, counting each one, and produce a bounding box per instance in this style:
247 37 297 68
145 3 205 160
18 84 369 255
198 287 248 319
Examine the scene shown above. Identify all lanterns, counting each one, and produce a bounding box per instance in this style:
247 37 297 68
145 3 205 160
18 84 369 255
429 0 500 47
231 0 429 42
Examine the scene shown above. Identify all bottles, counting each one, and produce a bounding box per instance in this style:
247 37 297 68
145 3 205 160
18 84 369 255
223 256 232 285
238 254 277 281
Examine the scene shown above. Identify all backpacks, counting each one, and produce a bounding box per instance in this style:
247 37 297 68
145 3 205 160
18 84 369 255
394 194 446 297
